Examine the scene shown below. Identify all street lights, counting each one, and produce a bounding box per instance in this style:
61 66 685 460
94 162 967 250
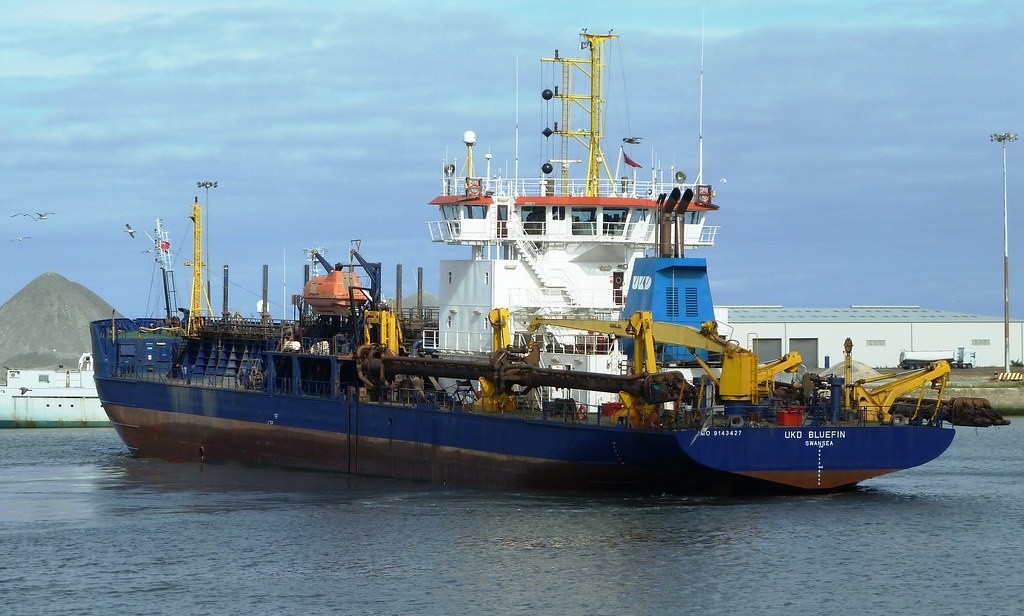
989 132 1020 373
197 181 218 316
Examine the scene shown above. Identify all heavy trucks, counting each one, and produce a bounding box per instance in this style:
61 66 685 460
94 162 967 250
897 344 978 369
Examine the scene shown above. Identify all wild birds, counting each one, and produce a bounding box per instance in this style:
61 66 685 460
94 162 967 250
11 236 31 241
36 212 55 219
11 213 37 221
123 224 135 238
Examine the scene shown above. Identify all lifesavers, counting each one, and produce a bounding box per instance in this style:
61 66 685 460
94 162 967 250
699 190 710 202
468 183 480 196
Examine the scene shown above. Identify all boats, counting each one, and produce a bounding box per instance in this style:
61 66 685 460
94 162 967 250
0 352 114 430
87 19 958 501
302 269 368 316
